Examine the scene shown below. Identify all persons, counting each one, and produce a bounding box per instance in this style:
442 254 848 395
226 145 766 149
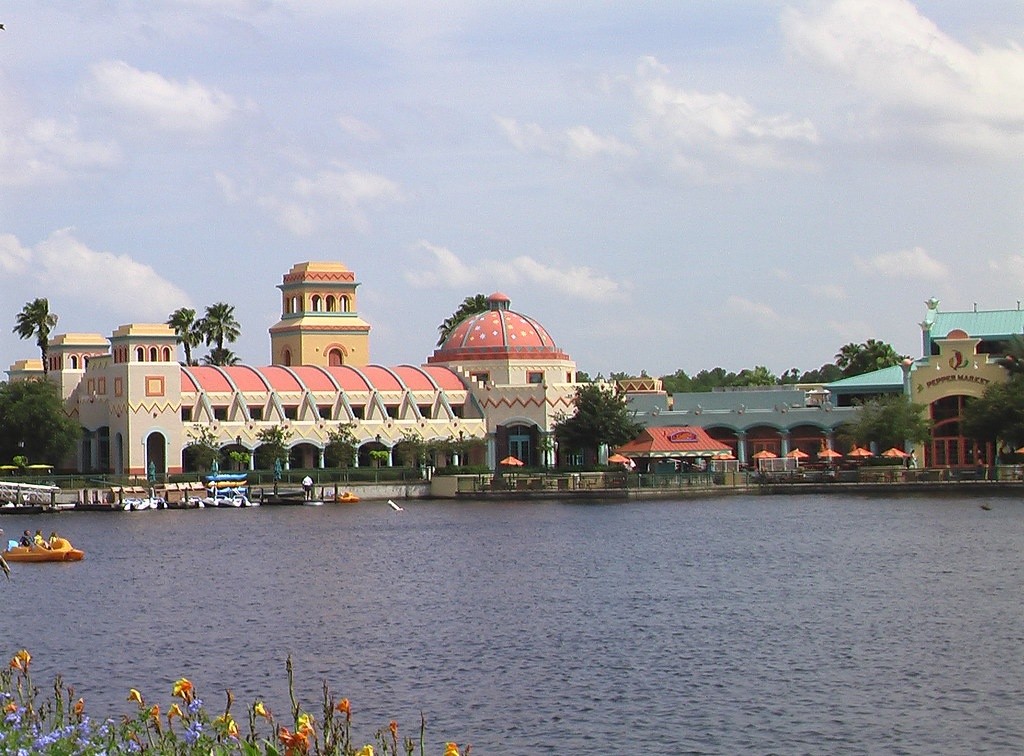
274 482 279 499
34 529 51 550
149 461 156 476
118 486 127 504
18 529 36 545
273 458 282 472
679 459 690 473
48 532 58 544
211 458 219 472
905 449 918 469
302 474 314 501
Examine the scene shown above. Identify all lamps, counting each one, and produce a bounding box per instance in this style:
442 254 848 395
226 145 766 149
737 403 746 414
775 402 789 413
973 361 979 369
823 400 833 413
652 405 659 416
694 404 702 415
936 361 941 372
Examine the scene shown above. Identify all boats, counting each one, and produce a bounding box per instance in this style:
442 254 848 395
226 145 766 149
122 494 252 510
0 482 75 514
337 493 359 502
0 538 84 562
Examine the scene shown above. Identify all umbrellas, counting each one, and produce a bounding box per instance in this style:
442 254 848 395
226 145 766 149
607 454 629 465
819 449 842 461
751 450 777 466
847 447 873 456
880 448 907 457
711 453 736 460
785 449 810 468
1014 447 1024 454
500 455 524 467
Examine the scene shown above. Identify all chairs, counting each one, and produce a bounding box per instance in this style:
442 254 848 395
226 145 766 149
557 478 571 491
491 478 547 491
